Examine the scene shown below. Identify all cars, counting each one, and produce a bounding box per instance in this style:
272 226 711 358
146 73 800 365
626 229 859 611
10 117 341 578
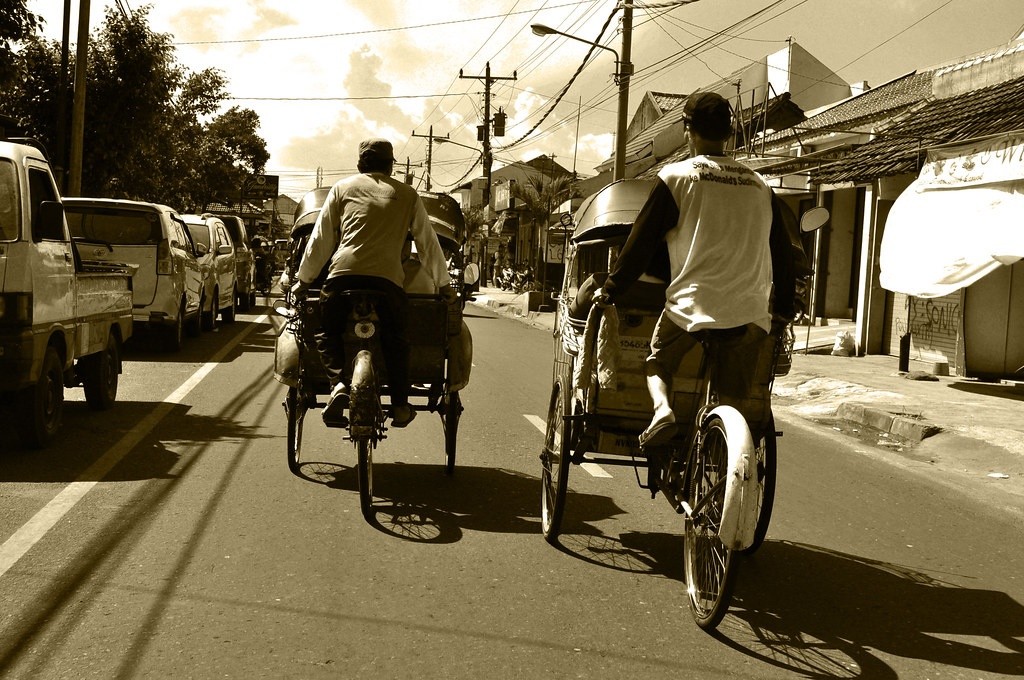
213 215 263 313
180 213 240 331
273 240 288 267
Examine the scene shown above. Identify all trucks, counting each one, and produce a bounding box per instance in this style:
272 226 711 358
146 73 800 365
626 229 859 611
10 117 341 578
0 139 134 448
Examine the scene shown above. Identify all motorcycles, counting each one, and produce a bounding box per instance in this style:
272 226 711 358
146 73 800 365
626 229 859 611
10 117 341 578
495 266 531 294
444 263 471 312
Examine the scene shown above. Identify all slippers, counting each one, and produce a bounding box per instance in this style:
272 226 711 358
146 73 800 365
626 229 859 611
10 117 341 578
322 393 350 422
391 410 417 427
638 422 677 450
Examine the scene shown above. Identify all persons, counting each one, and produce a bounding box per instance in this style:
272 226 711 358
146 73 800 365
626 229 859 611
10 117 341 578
493 244 505 288
295 138 457 428
591 92 798 448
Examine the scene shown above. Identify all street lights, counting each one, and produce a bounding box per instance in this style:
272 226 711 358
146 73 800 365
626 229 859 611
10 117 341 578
434 138 492 286
531 23 633 304
397 171 431 191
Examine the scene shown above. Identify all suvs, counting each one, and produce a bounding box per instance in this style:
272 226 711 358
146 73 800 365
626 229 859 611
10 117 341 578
61 195 207 351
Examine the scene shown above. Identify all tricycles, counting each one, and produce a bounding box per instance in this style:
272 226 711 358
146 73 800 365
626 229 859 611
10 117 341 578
541 179 781 629
272 187 472 514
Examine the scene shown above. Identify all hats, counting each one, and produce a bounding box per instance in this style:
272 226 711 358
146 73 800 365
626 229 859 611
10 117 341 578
683 92 730 122
359 141 397 163
